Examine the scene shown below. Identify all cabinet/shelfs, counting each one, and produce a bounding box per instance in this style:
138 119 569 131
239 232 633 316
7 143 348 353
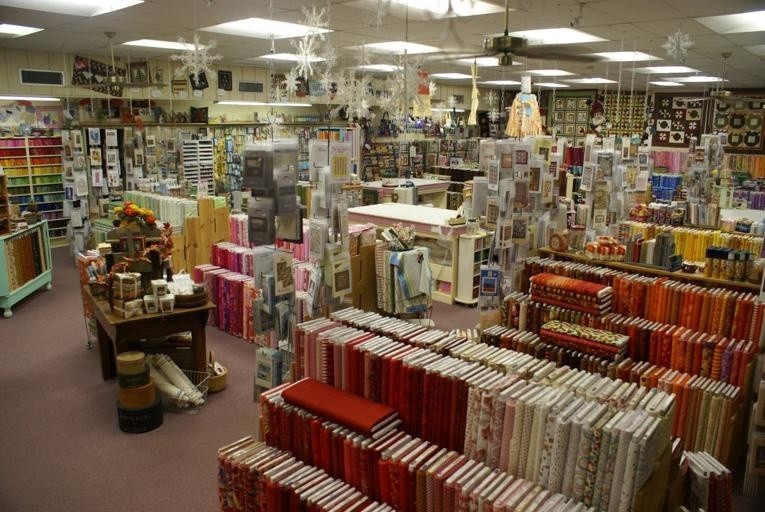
87 187 232 278
1 137 71 250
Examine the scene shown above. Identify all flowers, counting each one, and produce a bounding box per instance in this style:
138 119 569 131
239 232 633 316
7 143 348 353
110 201 157 232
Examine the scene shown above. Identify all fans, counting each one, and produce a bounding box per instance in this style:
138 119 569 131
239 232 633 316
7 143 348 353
59 29 170 94
677 50 765 109
430 1 608 82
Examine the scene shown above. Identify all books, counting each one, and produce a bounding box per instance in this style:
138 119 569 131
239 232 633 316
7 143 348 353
0 138 68 240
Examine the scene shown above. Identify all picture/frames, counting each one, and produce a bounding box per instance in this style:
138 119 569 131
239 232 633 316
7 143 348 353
119 236 145 252
36 106 62 129
217 69 233 90
124 59 154 87
552 94 589 124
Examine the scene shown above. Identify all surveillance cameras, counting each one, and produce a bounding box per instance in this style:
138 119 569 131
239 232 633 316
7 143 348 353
270 48 276 55
569 15 580 26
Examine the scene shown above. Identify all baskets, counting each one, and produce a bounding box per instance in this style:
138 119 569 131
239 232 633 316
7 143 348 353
209 349 228 393
154 370 211 416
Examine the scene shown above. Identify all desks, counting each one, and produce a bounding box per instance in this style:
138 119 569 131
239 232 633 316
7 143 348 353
84 268 218 389
